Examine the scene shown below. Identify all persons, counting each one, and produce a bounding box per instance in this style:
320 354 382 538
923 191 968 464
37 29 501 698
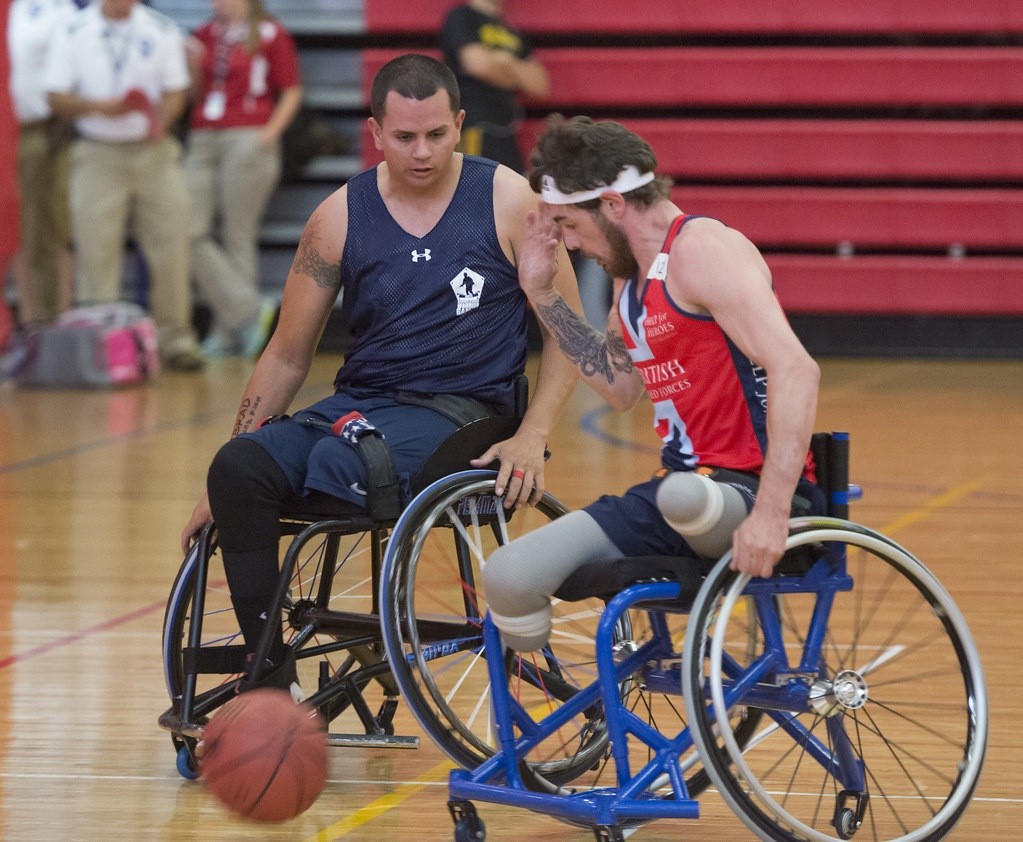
5 0 81 330
439 0 554 356
478 109 824 652
174 0 305 356
41 0 209 371
178 52 588 695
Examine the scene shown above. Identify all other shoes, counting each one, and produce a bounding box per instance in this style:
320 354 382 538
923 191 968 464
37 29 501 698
195 643 303 765
169 352 206 371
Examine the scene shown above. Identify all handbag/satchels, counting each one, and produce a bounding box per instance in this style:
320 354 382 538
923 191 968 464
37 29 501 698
1 299 159 390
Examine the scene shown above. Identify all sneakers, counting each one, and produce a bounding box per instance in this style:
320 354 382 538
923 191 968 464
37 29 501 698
200 336 240 358
240 297 281 360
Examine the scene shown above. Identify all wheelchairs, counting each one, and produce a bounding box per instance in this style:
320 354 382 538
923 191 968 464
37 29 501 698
375 430 989 841
157 372 550 784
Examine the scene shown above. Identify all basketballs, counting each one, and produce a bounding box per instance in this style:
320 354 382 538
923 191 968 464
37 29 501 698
200 686 331 824
